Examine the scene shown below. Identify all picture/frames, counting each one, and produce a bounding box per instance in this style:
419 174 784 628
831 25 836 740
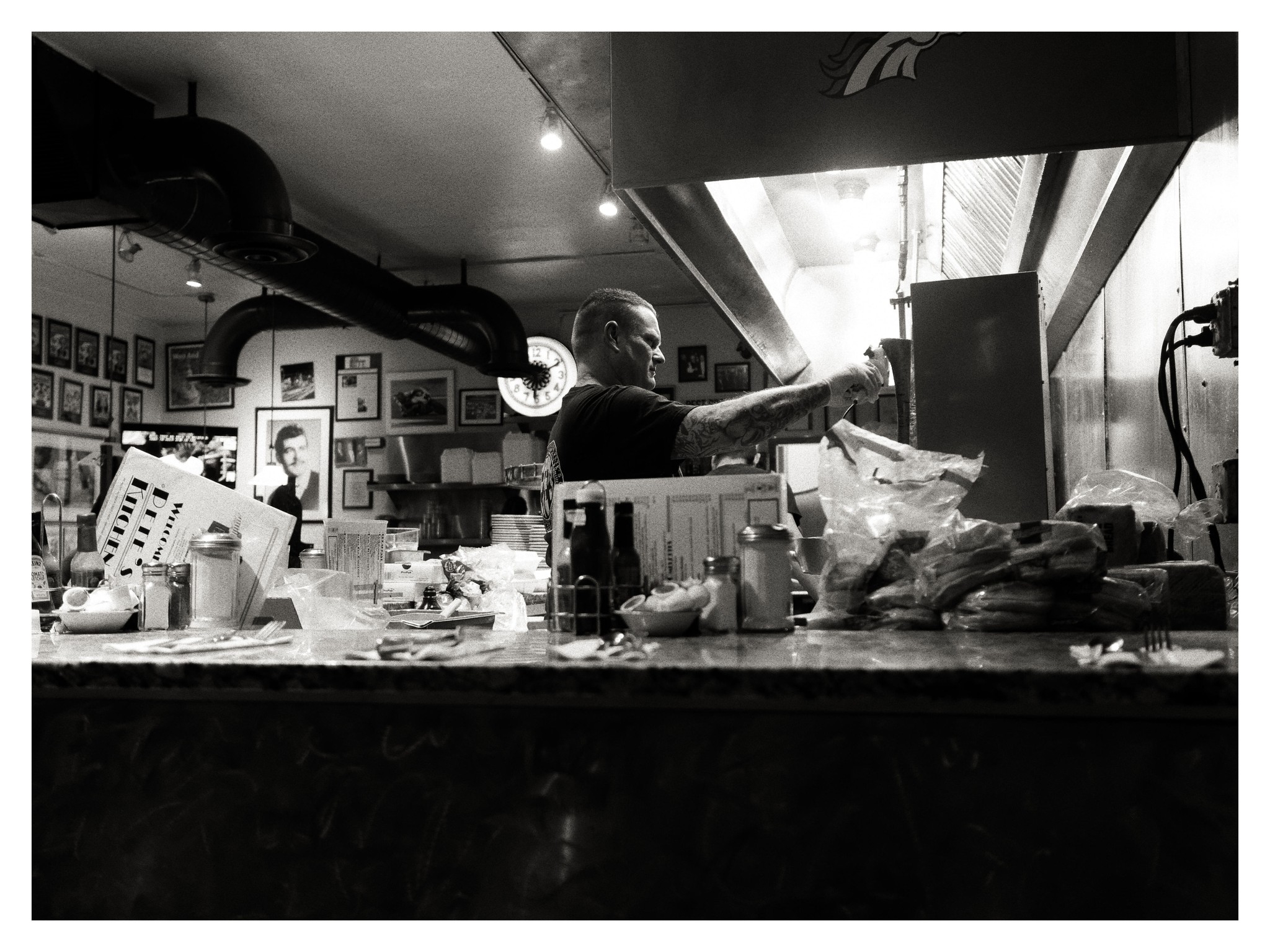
384 369 455 440
675 345 895 432
456 388 502 427
31 314 234 524
252 407 334 525
340 469 374 511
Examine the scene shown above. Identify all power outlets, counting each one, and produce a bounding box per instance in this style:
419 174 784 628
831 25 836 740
1210 457 1238 524
1211 280 1238 359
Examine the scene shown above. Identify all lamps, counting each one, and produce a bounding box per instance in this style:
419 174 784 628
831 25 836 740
627 219 651 246
598 181 619 217
184 258 204 290
115 228 145 266
538 102 567 151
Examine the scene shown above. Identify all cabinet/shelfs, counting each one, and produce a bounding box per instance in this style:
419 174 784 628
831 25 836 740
366 481 492 548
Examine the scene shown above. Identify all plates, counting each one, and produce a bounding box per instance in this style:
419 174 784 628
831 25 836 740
490 513 551 569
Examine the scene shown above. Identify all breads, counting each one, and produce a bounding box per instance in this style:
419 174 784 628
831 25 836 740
822 504 1229 630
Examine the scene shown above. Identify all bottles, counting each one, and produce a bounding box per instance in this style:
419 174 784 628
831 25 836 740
299 548 326 569
736 523 795 633
69 512 105 593
190 533 239 631
32 534 58 631
609 501 642 633
137 563 172 632
167 562 191 631
570 489 613 640
418 586 441 610
699 556 737 636
32 511 64 608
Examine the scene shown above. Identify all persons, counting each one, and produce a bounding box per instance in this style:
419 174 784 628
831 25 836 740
704 444 804 538
267 422 319 511
540 288 884 620
679 352 705 379
158 440 204 476
31 333 138 423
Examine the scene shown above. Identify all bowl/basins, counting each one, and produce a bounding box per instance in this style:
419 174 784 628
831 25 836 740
612 607 701 637
52 608 138 633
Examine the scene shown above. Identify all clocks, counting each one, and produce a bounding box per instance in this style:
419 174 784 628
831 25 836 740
497 335 577 419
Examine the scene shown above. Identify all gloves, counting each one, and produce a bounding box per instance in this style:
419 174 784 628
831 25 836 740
822 359 884 408
